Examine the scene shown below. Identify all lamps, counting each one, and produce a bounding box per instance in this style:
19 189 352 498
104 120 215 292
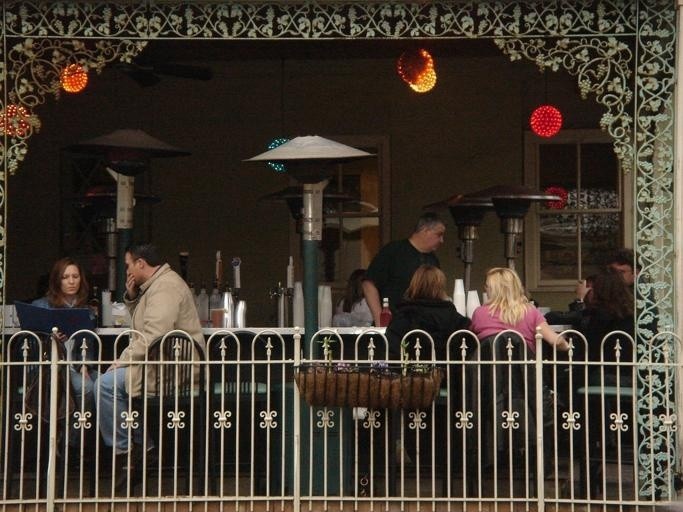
397 45 438 93
267 40 298 174
544 145 568 210
528 67 563 138
0 63 88 137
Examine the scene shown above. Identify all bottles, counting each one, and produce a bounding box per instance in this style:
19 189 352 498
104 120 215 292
287 255 296 289
198 289 222 322
90 286 101 328
380 298 393 327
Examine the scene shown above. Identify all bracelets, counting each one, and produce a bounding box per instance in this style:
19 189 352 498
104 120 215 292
574 298 584 304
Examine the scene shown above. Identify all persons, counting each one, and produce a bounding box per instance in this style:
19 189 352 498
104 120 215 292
465 265 573 477
359 212 450 325
563 265 634 454
606 246 659 330
22 256 105 483
379 263 470 465
90 238 207 494
330 267 373 325
566 273 598 319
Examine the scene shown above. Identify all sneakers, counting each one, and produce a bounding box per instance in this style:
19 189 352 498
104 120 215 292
45 441 170 492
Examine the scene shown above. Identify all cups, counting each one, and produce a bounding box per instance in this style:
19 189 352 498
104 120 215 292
211 309 223 327
453 279 481 320
318 285 333 328
293 280 305 328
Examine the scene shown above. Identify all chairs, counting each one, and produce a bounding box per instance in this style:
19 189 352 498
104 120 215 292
0 330 633 499
